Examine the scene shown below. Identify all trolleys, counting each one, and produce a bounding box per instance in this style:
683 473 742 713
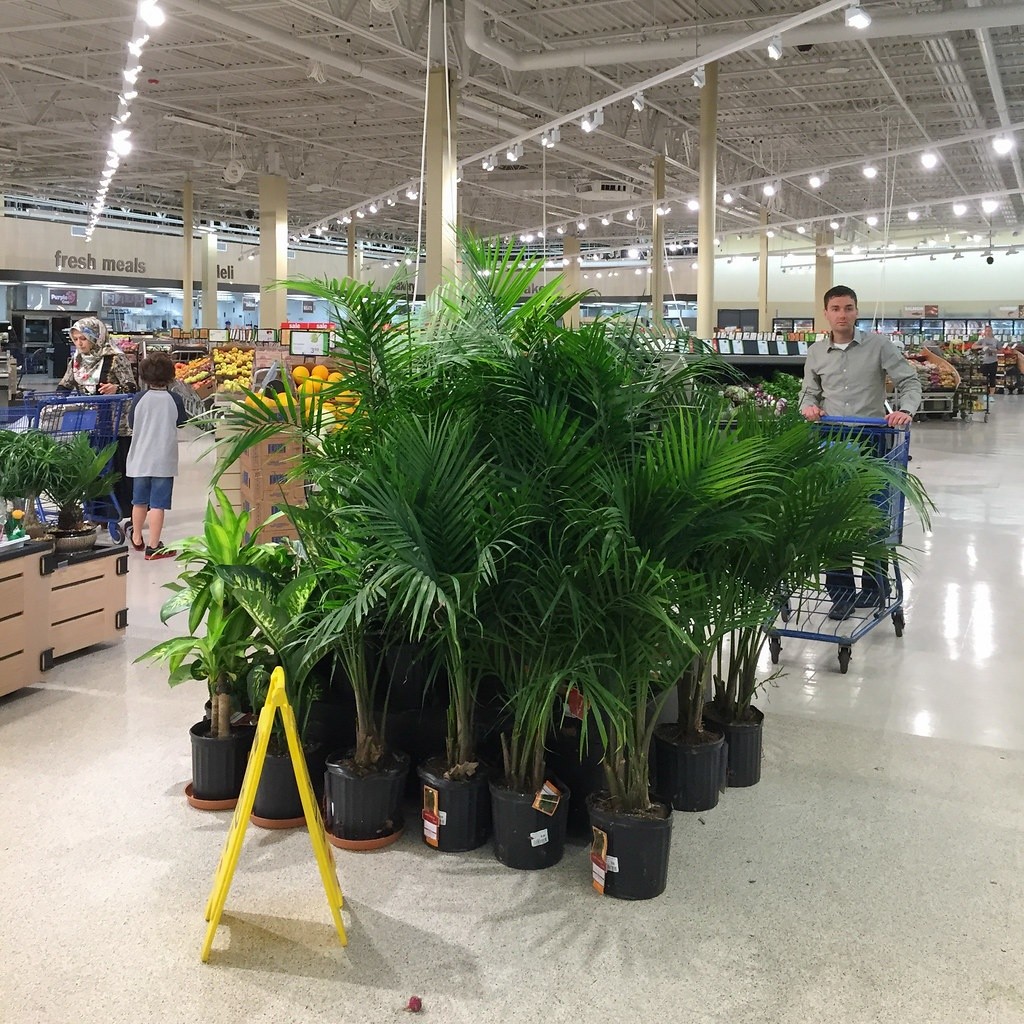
761 416 912 674
954 363 991 422
0 387 136 546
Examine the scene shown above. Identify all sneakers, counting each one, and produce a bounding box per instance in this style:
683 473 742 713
125 520 145 550
856 587 892 607
828 601 854 620
146 541 177 560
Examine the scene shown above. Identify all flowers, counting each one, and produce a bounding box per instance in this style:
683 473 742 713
4 510 26 542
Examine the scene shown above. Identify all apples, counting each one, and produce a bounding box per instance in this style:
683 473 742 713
213 347 254 391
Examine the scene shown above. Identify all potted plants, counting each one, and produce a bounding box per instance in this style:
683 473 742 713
0 427 121 557
130 226 939 897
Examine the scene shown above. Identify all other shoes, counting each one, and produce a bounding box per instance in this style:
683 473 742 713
989 397 995 402
983 395 987 400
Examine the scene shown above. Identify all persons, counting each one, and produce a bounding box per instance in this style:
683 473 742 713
799 285 922 620
972 326 1004 402
57 318 140 531
126 351 188 560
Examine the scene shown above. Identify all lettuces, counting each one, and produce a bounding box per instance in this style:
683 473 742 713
747 383 787 416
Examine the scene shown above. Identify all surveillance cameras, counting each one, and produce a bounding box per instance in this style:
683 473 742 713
987 257 994 264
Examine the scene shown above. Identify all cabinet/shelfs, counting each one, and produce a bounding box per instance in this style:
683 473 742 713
887 340 1024 422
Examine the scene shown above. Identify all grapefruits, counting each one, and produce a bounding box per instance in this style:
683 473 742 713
245 364 362 433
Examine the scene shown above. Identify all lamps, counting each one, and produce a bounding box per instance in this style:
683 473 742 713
84 0 1024 281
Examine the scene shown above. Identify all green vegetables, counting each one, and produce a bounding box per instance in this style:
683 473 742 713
758 372 803 413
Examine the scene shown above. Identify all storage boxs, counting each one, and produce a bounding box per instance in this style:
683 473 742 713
180 361 212 381
245 406 303 437
241 432 302 469
255 350 281 369
209 344 255 379
240 463 306 501
290 362 341 404
191 377 217 400
241 501 305 530
242 530 300 546
